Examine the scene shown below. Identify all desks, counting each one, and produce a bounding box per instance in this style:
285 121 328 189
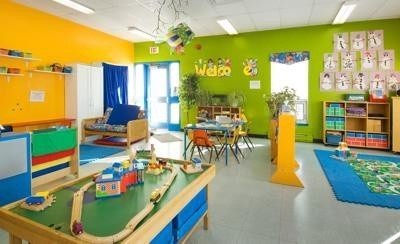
1 156 215 244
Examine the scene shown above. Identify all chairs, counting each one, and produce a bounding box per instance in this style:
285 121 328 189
81 107 149 149
182 120 256 167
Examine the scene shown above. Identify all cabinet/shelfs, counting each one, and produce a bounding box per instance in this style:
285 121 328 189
322 100 390 151
195 106 241 122
64 63 104 120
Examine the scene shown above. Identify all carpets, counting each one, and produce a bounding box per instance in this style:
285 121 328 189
79 144 126 167
153 132 182 143
314 149 399 211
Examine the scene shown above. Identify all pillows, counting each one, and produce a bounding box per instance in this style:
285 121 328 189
106 104 140 126
